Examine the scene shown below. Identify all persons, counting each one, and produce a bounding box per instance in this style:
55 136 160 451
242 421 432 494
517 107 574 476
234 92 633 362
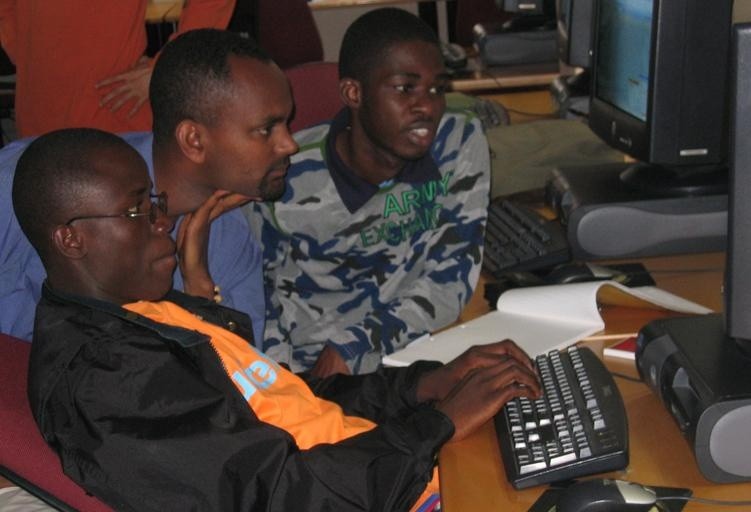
1 0 240 136
246 9 495 387
12 127 545 512
1 24 299 359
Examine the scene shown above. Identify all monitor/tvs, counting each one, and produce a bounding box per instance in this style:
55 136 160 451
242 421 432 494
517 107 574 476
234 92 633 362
588 0 735 194
720 21 751 359
555 0 593 97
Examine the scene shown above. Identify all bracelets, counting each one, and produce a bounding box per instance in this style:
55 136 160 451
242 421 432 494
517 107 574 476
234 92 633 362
212 284 223 305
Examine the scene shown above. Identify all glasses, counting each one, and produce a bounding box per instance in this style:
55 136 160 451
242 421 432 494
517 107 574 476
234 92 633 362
63 191 170 226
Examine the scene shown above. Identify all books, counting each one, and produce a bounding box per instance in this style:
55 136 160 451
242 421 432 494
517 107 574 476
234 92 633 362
382 278 717 368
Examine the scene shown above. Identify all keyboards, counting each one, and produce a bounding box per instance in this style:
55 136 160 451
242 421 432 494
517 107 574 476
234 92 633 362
480 196 572 279
492 343 631 492
469 98 511 131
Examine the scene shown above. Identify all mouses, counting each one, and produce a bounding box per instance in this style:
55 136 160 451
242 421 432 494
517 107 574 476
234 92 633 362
554 476 658 512
545 261 613 285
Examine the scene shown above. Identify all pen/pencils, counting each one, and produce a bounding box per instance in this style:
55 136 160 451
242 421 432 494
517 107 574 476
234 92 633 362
583 333 638 342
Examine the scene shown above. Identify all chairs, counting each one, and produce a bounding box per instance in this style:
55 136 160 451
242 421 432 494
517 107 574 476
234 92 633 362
283 61 344 130
0 332 114 512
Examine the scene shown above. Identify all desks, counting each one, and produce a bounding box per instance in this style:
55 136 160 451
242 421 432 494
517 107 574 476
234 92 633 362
438 91 751 512
